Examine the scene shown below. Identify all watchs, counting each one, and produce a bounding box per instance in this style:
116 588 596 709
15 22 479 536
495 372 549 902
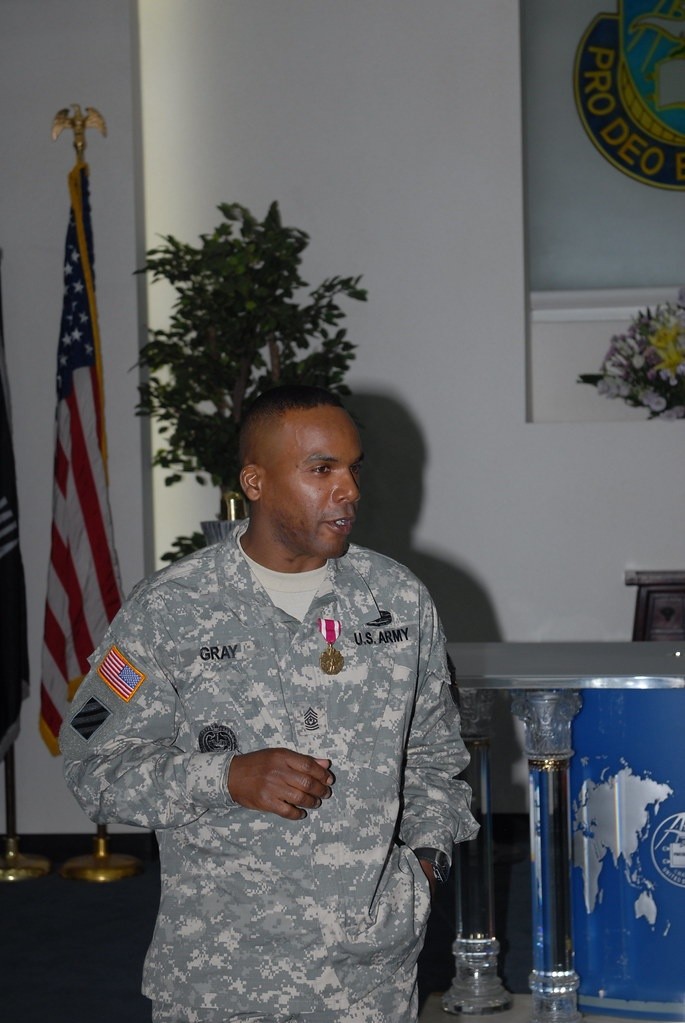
413 847 452 885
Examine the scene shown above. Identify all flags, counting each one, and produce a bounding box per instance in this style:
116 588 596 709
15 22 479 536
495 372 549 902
0 250 32 765
42 152 124 757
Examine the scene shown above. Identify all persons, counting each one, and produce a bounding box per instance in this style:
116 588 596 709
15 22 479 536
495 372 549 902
56 379 480 1023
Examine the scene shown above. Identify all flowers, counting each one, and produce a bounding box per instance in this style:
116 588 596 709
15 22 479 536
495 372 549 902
576 292 685 423
